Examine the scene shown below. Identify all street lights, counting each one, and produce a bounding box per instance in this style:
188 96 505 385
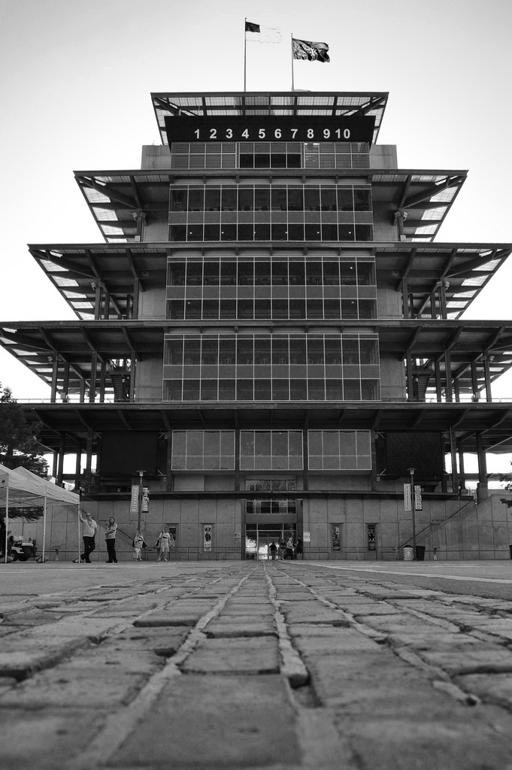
405 464 417 560
136 469 147 534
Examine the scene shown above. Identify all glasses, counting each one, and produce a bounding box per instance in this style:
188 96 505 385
86 515 91 517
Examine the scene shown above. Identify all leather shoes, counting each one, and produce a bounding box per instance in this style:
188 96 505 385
105 560 118 563
81 554 91 563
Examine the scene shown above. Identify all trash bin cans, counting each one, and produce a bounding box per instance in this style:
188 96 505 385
403 545 426 561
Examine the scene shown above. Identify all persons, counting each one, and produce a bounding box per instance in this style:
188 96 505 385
80 512 98 563
159 527 171 562
104 517 117 563
439 467 448 492
134 531 144 561
269 535 303 560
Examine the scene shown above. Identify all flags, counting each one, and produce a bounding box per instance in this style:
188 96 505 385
245 22 260 32
292 38 330 62
